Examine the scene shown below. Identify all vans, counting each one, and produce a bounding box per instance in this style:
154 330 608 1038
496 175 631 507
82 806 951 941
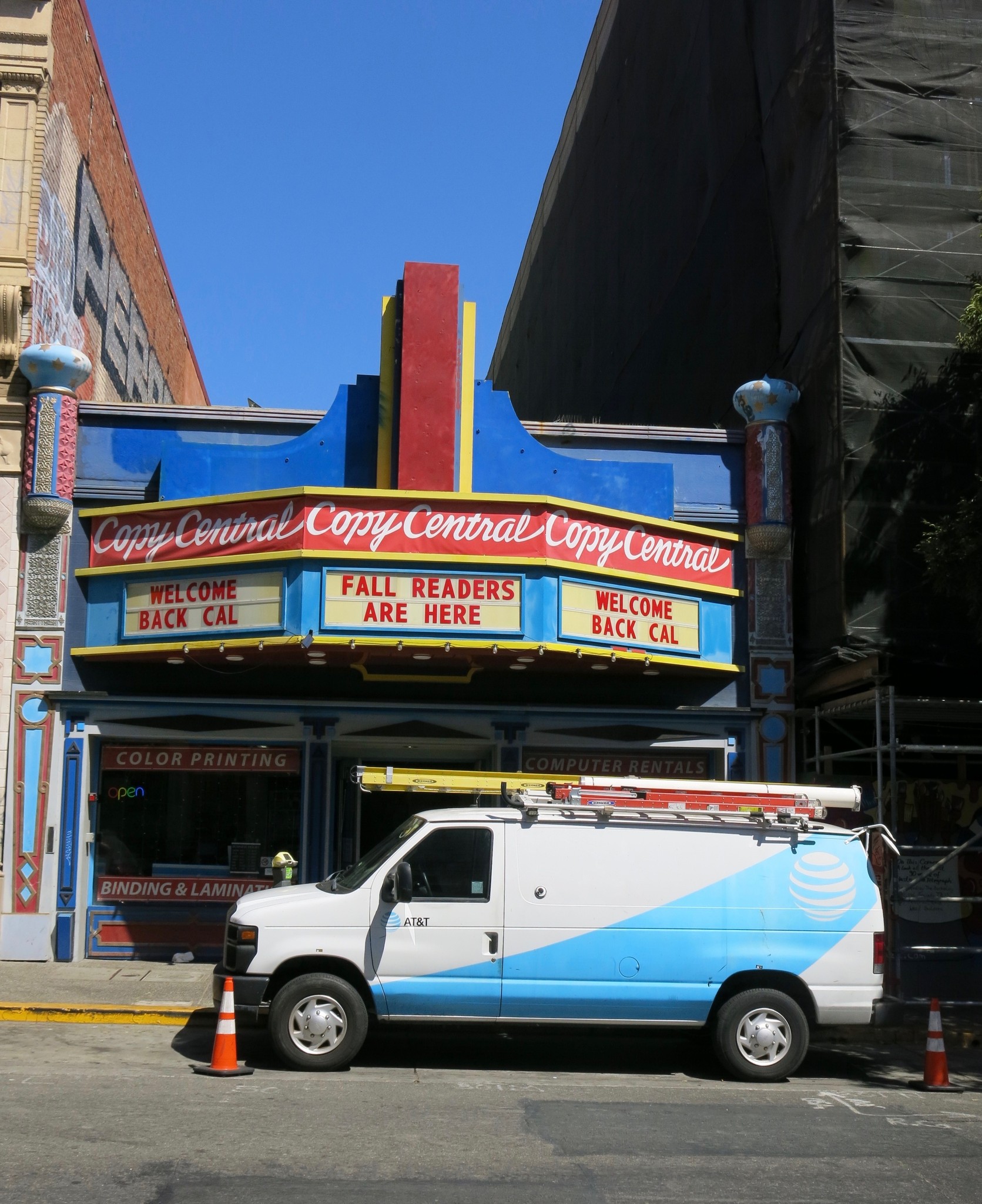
215 780 898 1084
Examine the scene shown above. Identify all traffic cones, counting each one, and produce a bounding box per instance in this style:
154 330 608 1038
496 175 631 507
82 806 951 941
907 997 965 1093
192 977 255 1078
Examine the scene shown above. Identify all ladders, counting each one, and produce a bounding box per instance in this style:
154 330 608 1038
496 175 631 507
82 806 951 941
543 781 827 820
349 765 863 811
